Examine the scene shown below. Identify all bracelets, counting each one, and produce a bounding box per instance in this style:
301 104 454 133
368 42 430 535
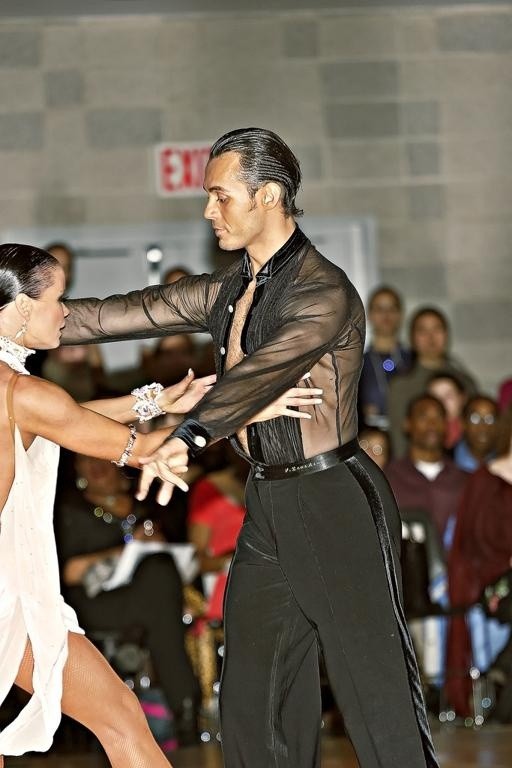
128 381 167 428
114 421 135 468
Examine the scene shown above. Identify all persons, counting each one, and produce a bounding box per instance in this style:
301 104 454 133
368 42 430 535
57 127 437 768
1 239 323 768
38 246 511 747
